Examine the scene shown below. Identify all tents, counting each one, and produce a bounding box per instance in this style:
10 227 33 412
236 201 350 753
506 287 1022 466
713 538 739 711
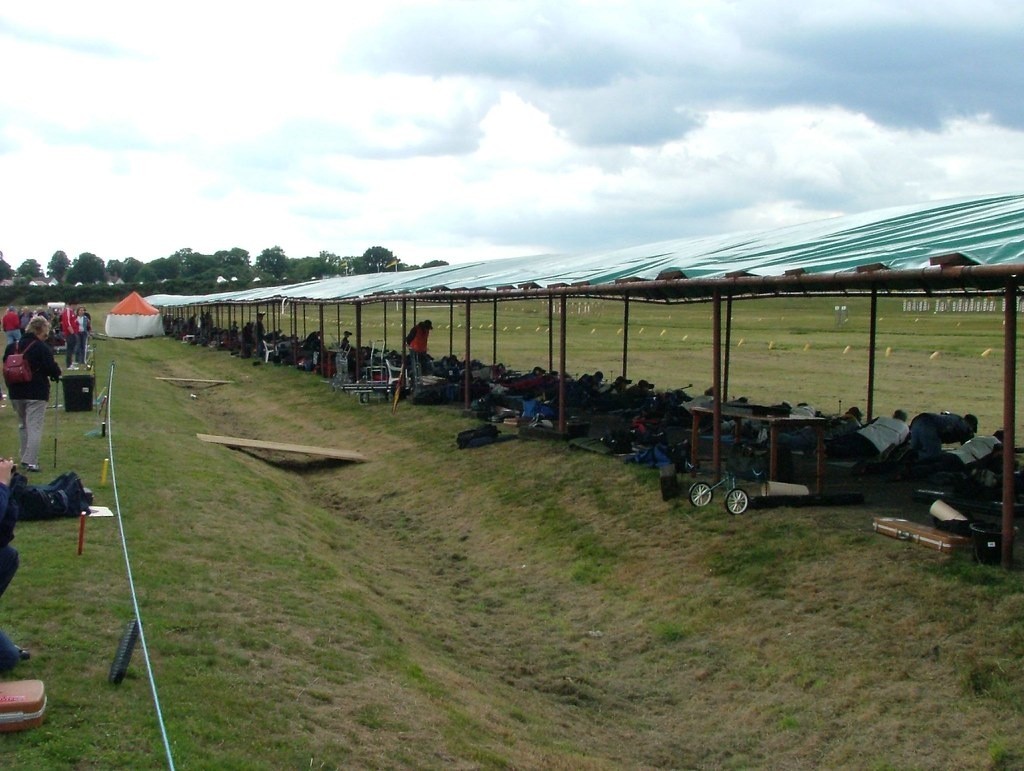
105 292 166 339
143 194 1024 570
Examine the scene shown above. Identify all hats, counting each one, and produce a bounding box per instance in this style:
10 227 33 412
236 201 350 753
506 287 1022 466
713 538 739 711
674 389 694 402
9 305 16 311
534 366 546 374
638 380 655 389
615 376 633 385
344 331 352 335
426 354 434 360
422 319 433 329
965 414 978 433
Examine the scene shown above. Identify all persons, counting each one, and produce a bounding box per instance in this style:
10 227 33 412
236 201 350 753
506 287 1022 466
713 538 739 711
1 459 29 671
3 307 21 344
75 306 92 364
814 409 909 462
886 430 1004 485
189 314 197 328
406 320 433 375
4 316 62 472
253 313 266 360
62 300 79 370
19 306 64 345
881 412 978 465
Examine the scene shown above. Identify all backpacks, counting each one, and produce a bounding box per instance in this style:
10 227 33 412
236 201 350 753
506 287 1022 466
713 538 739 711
3 339 38 384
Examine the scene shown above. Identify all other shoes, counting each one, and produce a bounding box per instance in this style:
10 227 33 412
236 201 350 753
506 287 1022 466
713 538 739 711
15 645 31 661
20 462 42 472
67 361 79 371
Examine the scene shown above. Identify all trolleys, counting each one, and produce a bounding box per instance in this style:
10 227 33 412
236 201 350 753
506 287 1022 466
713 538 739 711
343 340 411 404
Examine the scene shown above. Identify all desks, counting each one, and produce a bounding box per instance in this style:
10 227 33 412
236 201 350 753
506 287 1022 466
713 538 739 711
686 405 827 495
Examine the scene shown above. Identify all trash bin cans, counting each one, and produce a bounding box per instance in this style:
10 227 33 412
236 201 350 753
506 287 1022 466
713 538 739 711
59 374 97 413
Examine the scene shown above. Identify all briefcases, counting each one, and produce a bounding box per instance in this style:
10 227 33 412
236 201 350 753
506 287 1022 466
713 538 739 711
0 679 47 734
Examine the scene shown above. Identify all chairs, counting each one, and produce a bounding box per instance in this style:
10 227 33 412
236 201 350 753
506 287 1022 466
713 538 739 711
263 340 274 363
385 358 410 388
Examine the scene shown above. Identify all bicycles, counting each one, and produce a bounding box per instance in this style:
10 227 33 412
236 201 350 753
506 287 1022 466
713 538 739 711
689 468 748 516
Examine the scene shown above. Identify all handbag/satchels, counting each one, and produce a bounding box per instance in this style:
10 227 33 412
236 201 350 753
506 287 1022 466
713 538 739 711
11 472 92 522
